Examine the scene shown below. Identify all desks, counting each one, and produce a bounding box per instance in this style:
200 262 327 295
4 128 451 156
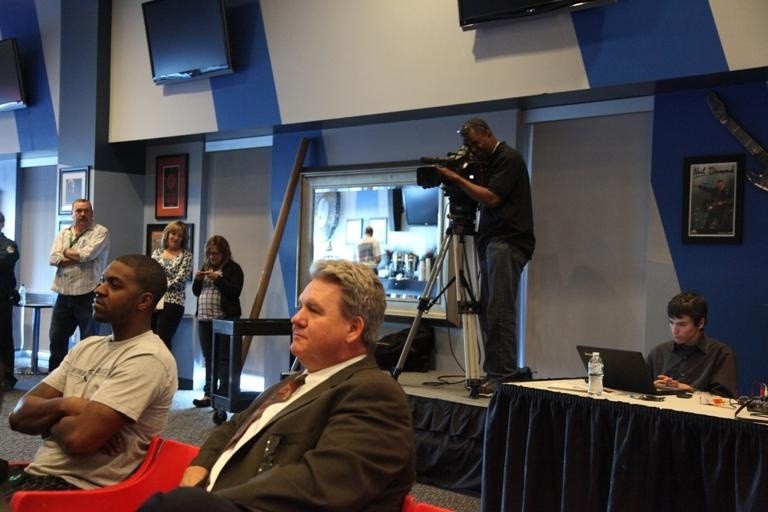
481 377 768 512
13 303 56 374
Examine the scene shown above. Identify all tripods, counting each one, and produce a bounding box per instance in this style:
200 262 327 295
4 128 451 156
392 199 484 391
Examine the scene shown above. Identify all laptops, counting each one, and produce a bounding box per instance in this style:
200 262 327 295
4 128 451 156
577 345 693 395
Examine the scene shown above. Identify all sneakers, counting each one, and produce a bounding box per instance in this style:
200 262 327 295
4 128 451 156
477 381 497 397
193 398 211 407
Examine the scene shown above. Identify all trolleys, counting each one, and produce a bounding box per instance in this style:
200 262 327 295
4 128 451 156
209 317 301 426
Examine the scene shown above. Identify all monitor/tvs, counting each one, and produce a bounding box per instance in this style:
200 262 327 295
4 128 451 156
141 0 234 86
459 0 590 26
0 37 27 112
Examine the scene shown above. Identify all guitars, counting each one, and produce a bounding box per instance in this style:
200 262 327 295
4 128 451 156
710 94 768 192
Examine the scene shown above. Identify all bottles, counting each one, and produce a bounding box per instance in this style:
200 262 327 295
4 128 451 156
18 284 26 306
588 352 604 395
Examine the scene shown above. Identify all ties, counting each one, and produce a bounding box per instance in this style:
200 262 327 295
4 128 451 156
225 374 305 451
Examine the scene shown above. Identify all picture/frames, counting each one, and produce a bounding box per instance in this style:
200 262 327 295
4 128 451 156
155 153 188 218
146 224 193 283
681 154 745 243
58 221 73 231
58 165 90 215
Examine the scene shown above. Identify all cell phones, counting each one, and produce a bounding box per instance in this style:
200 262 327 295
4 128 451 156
200 272 209 275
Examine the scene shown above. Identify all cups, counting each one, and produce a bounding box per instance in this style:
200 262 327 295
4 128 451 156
418 258 431 283
394 251 405 274
406 253 418 277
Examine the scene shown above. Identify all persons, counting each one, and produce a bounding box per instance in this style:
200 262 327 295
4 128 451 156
48 198 111 373
357 225 381 275
151 220 192 349
0 210 18 393
436 115 536 397
135 486 240 512
644 290 736 398
193 235 243 407
692 179 725 229
182 259 417 512
0 253 178 500
